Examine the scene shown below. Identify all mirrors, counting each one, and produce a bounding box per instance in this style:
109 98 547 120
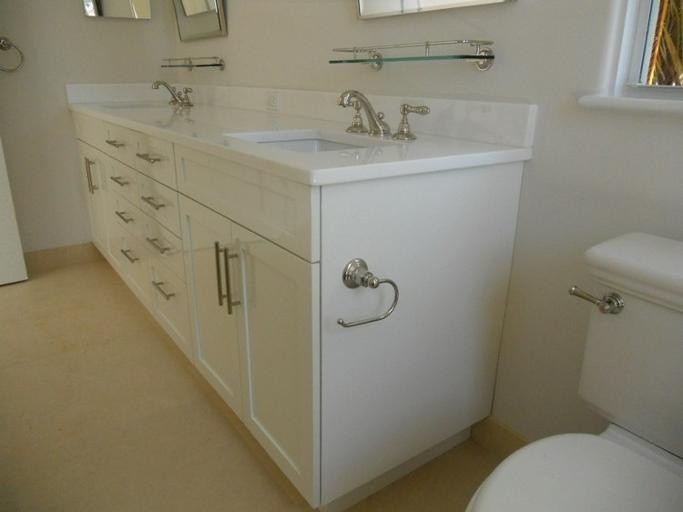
355 1 513 20
83 0 150 21
174 0 229 44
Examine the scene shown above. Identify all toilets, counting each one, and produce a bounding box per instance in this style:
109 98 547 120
463 233 682 512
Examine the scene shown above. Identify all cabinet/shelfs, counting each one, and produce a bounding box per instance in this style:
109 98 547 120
70 111 112 275
107 120 196 366
179 159 526 510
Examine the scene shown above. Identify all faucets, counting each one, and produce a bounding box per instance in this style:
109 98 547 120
151 79 194 107
337 89 390 137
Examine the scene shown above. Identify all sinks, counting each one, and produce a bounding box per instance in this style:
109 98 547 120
223 128 400 153
99 103 162 111
